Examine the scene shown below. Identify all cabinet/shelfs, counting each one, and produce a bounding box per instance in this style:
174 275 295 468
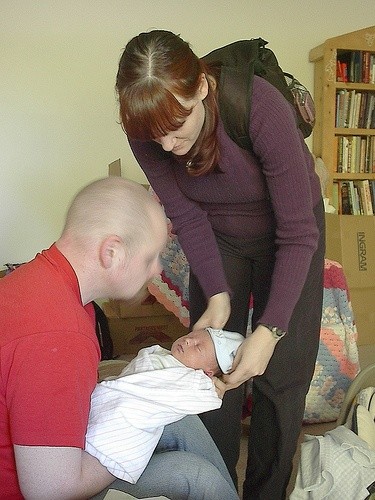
307 26 375 214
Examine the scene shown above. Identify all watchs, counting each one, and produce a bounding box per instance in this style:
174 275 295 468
263 324 286 338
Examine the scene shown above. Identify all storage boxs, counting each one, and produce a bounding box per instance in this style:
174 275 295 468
102 301 189 355
324 214 375 346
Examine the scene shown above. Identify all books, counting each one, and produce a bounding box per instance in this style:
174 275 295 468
335 49 375 215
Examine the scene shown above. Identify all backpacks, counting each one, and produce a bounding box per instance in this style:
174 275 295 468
200 39 318 150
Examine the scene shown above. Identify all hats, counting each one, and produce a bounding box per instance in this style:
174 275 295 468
205 328 245 375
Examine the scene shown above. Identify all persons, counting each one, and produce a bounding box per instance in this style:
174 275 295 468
116 30 326 500
0 177 240 500
86 327 245 484
